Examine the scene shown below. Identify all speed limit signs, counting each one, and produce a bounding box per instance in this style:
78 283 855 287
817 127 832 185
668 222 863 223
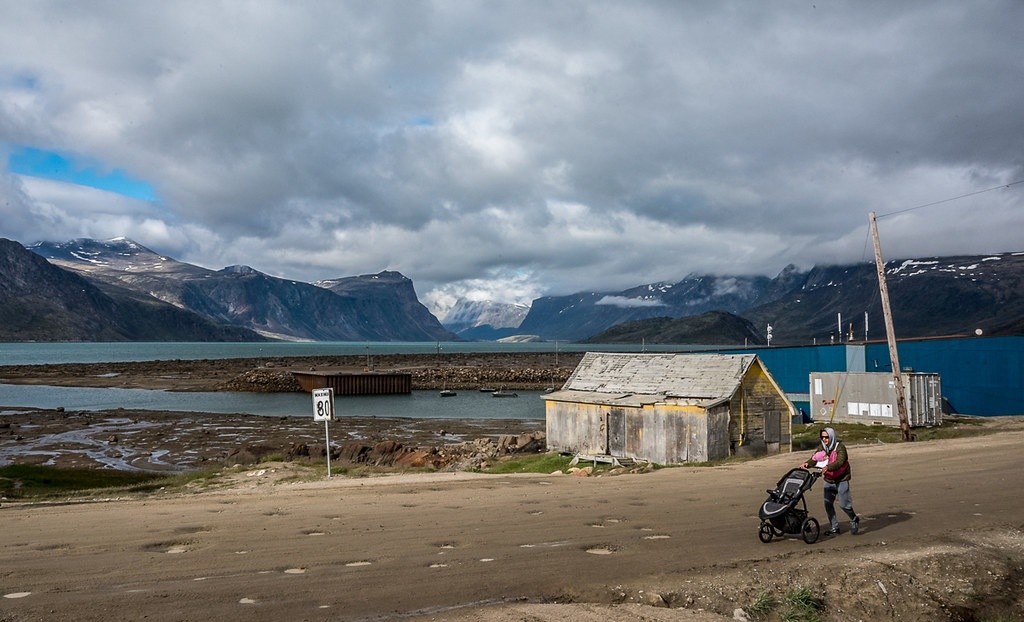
312 388 336 422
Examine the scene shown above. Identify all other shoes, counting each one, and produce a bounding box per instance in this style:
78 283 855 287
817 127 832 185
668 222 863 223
824 528 841 536
849 515 859 535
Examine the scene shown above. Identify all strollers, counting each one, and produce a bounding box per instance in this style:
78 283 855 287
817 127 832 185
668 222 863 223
757 465 824 545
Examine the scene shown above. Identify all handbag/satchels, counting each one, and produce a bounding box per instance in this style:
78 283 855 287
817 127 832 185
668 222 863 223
822 442 850 480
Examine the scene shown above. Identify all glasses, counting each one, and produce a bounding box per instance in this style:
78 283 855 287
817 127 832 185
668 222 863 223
820 436 829 440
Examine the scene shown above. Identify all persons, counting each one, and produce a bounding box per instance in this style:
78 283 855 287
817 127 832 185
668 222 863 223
799 427 859 536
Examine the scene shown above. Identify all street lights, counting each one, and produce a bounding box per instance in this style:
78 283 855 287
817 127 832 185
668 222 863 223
435 341 442 366
260 348 264 367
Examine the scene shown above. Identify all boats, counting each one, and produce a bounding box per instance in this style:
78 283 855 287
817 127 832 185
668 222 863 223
493 385 518 398
545 380 560 393
479 386 496 393
440 390 457 398
